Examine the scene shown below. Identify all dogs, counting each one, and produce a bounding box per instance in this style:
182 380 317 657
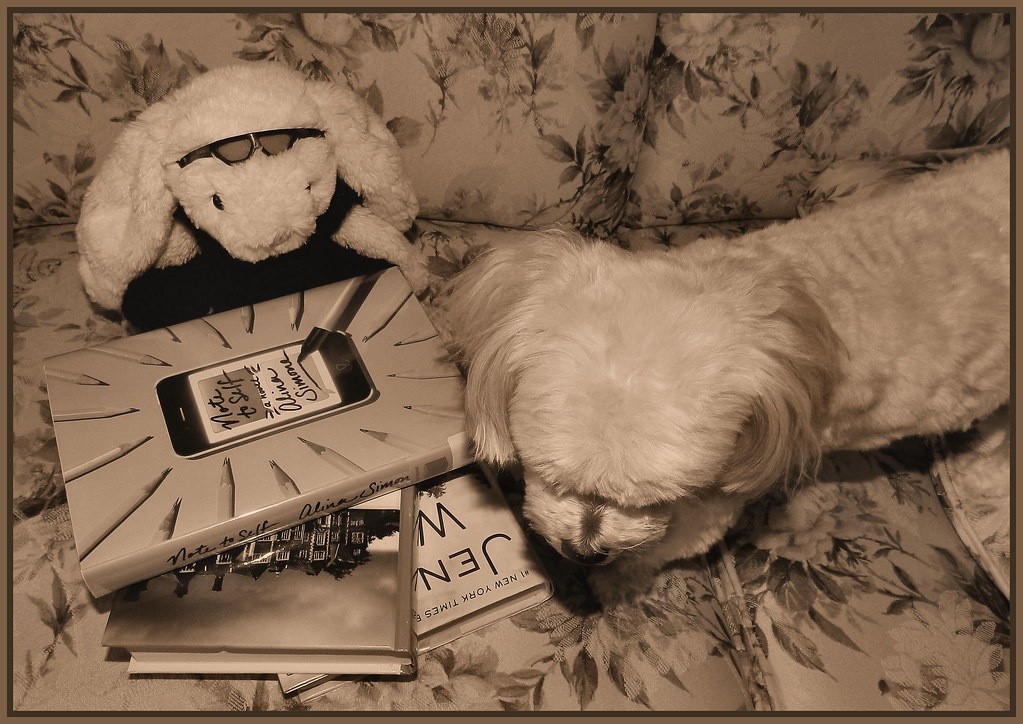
428 146 1009 571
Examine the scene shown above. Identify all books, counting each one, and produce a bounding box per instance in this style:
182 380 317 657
42 264 480 599
278 462 554 706
97 486 419 679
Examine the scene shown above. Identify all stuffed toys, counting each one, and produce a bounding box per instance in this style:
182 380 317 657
78 62 435 310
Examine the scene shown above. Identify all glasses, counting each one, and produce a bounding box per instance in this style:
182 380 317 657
178 128 325 167
